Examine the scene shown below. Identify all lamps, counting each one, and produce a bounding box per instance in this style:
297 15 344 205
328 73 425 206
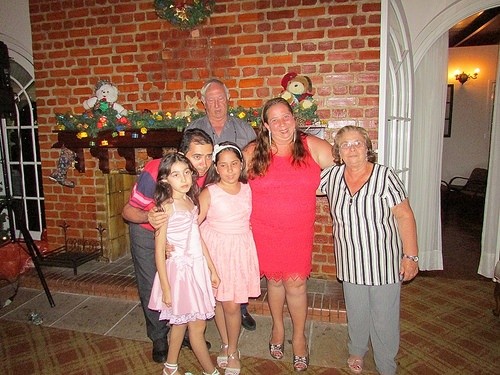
455 68 479 85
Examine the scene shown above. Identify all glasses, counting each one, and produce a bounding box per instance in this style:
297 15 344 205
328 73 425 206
337 139 367 150
202 95 227 104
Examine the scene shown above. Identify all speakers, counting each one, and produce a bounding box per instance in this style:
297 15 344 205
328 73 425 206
0 41 14 115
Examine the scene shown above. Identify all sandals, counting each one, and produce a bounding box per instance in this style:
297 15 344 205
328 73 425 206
224 349 242 375
216 343 229 370
202 367 220 375
162 362 182 375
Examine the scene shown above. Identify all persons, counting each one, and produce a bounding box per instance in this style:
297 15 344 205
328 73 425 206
241 98 337 371
316 126 419 375
198 141 261 375
121 128 214 363
148 152 221 375
184 79 258 331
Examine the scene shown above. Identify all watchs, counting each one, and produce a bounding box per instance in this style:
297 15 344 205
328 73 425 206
402 253 419 262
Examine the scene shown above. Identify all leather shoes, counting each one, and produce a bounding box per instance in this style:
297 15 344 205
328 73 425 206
241 313 257 331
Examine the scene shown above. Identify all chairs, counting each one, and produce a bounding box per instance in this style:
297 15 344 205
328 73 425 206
442 168 488 225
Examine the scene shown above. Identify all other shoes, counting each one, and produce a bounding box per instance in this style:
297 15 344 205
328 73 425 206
183 338 211 351
150 337 169 362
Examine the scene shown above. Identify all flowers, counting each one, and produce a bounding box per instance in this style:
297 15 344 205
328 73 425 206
54 106 328 146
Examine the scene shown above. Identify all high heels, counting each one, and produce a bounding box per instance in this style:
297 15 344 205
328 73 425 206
268 325 285 359
346 354 364 374
292 335 309 372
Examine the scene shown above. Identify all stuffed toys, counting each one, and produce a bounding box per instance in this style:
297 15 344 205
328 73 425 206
83 79 128 117
280 72 316 108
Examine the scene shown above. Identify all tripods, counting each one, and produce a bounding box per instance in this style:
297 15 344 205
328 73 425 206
0 115 55 308
